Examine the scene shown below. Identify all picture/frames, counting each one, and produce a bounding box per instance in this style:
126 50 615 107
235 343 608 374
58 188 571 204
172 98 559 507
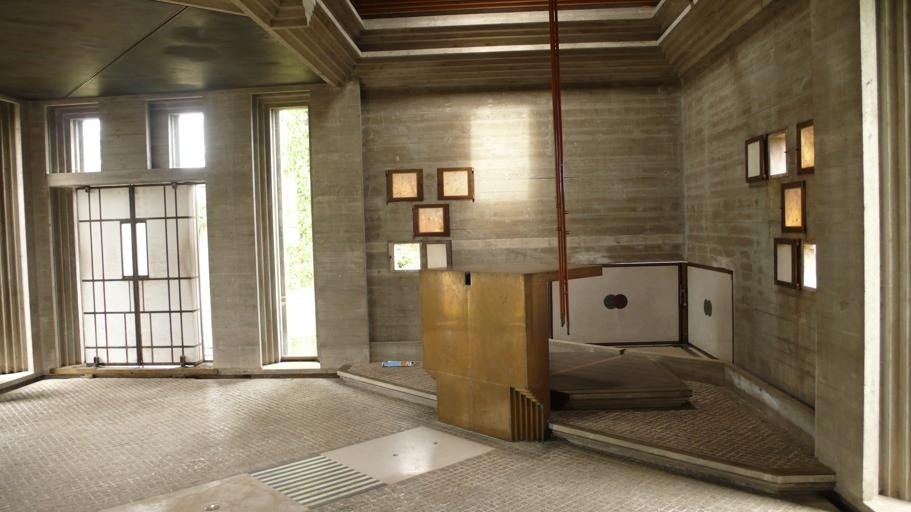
744 119 818 294
384 166 476 271
